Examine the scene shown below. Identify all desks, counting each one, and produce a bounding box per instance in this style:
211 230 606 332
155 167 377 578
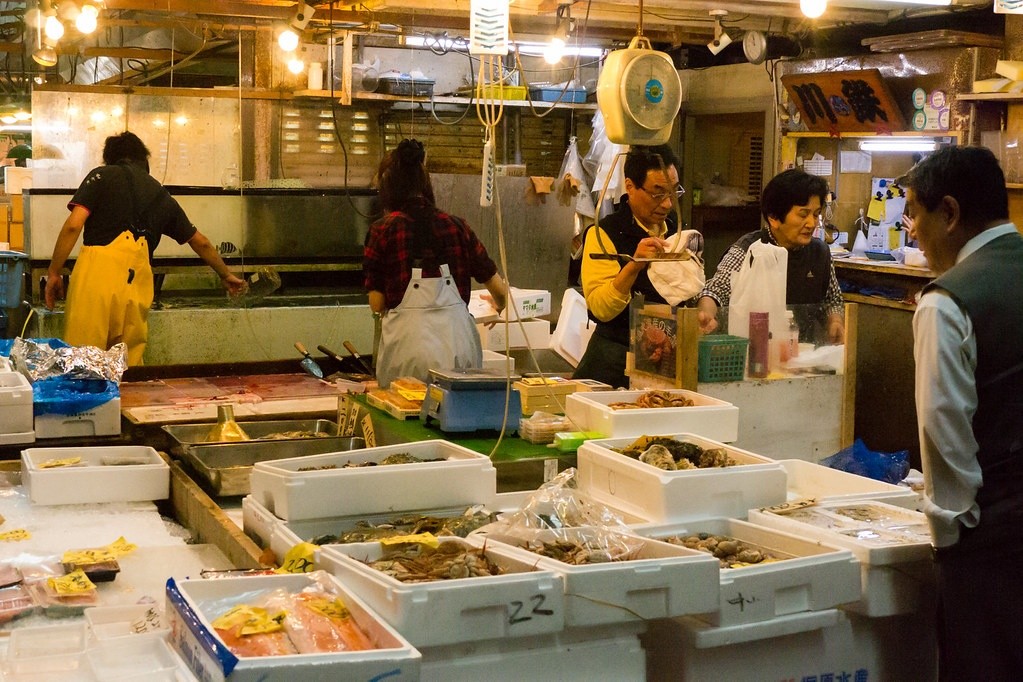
691 204 763 259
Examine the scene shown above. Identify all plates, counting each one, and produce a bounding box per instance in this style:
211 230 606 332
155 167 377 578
866 250 895 261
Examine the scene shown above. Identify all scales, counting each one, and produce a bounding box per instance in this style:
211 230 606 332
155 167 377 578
588 39 692 262
420 368 520 438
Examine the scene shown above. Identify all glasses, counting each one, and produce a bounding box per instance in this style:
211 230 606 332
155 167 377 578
632 179 686 202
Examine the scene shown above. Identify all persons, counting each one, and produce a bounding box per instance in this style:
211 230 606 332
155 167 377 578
46 131 249 366
895 143 1023 682
365 149 508 389
572 144 694 388
899 214 917 240
692 169 844 346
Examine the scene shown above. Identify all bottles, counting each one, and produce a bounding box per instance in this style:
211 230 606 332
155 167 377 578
787 310 799 357
308 63 323 90
236 267 281 301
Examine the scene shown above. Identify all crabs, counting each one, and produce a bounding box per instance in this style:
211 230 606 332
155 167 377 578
639 324 672 361
297 390 764 580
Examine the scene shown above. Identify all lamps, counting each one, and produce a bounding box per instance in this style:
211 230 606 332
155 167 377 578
272 20 304 74
30 38 59 67
856 138 940 152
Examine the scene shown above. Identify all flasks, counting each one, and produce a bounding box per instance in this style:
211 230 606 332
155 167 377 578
748 310 769 379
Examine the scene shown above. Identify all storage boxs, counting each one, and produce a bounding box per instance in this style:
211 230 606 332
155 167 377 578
0 286 933 682
457 84 528 101
526 88 588 102
362 77 435 96
0 250 28 308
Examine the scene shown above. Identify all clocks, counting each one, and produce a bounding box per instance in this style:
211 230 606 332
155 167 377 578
743 29 766 65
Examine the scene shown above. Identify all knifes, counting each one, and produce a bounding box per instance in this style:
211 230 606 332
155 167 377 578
317 344 366 375
295 341 323 379
343 341 375 376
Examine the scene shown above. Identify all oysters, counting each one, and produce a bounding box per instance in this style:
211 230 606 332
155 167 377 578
609 432 746 473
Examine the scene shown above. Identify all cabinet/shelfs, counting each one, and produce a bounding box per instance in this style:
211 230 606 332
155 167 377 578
783 96 1023 471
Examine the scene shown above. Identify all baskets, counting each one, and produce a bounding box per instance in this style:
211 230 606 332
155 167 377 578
697 335 750 384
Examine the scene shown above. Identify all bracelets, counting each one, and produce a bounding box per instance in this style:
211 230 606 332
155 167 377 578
931 546 954 562
